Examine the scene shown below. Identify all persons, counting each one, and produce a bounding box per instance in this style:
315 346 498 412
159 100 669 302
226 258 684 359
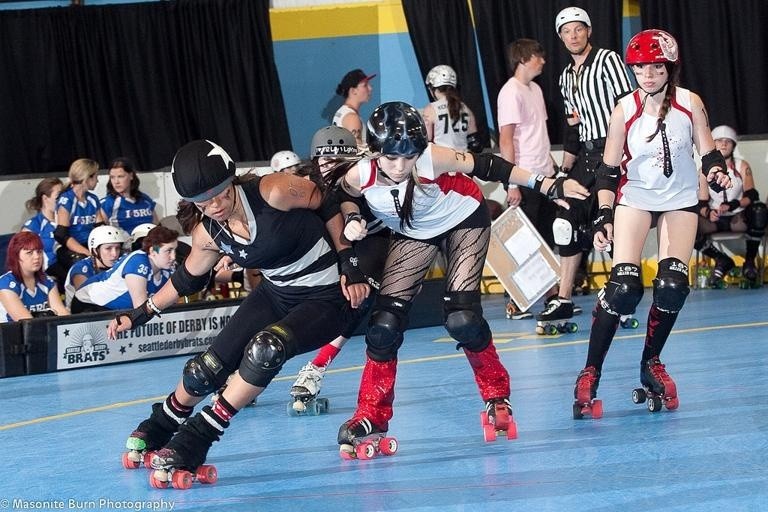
104 136 376 489
569 28 733 421
211 126 394 416
300 100 590 459
692 124 766 290
420 64 482 180
330 68 376 144
496 38 582 320
1 147 340 320
532 4 636 336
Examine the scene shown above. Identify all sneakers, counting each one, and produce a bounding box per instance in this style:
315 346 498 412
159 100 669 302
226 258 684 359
505 302 535 321
543 294 584 316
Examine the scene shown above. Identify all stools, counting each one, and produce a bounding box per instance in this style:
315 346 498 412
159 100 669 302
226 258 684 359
695 232 765 289
576 244 612 293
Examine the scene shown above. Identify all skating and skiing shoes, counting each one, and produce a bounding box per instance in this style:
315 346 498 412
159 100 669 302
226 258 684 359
286 362 331 417
143 410 219 489
631 357 680 412
617 307 640 328
120 400 188 470
478 392 519 442
572 368 604 421
704 254 739 289
335 412 399 461
738 263 763 289
532 298 579 334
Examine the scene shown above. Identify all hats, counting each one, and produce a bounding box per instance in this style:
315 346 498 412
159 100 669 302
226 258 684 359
338 67 377 90
168 140 238 203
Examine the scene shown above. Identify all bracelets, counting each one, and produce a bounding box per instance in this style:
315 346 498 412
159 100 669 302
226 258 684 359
506 185 518 189
528 172 537 190
533 175 546 192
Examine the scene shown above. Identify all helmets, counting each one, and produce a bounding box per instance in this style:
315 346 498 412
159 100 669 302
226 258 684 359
425 64 458 89
88 224 129 270
129 222 160 244
269 149 302 172
711 124 739 147
310 123 358 159
624 28 680 68
365 101 429 159
554 7 594 37
